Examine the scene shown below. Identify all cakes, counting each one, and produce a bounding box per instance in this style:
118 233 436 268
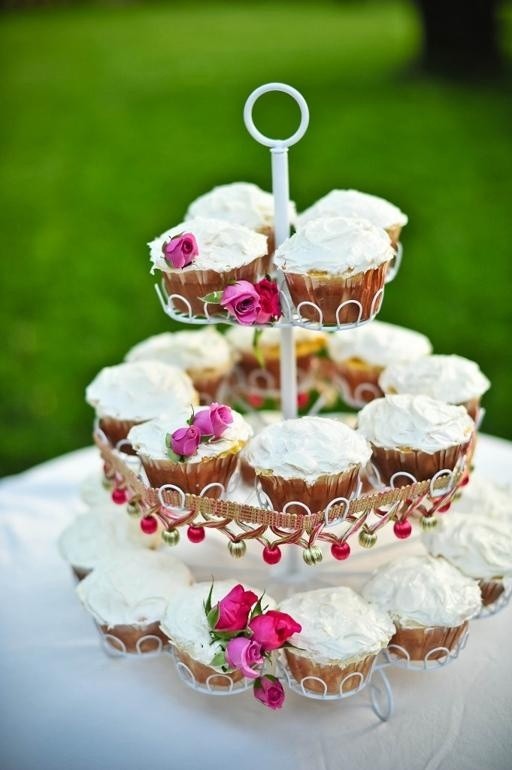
294 188 409 275
147 217 269 316
273 216 394 323
186 182 296 273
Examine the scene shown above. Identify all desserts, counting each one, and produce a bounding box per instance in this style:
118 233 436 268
57 314 512 692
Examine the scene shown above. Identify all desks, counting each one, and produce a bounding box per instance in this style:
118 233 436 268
0 433 512 770
